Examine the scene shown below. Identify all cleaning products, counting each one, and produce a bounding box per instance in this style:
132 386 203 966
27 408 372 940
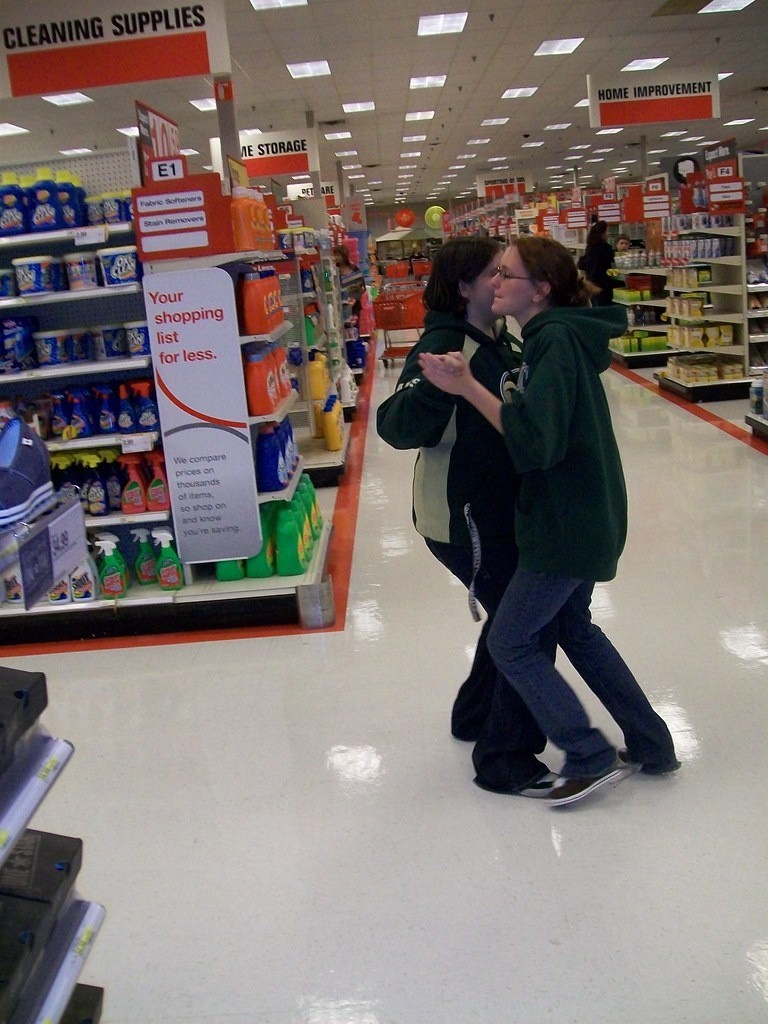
0 165 378 611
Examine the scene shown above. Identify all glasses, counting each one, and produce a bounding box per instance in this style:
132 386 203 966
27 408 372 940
497 265 531 280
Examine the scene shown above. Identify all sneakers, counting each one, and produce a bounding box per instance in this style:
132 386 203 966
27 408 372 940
513 772 571 798
544 754 625 806
618 748 681 775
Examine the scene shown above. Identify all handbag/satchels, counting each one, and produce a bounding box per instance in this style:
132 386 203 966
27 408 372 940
577 254 586 270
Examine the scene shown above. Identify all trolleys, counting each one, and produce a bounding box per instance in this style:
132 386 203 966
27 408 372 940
372 279 429 369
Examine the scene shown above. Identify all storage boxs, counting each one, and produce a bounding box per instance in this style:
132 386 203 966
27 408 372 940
608 268 743 383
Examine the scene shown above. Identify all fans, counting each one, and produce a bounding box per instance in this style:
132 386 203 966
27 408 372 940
424 206 447 229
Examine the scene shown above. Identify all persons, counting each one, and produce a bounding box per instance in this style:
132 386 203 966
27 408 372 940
335 245 367 341
582 220 630 307
419 238 679 809
408 251 415 275
376 238 560 798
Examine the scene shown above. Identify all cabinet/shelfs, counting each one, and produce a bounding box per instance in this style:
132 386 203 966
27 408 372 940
608 267 675 370
0 223 178 646
0 522 105 1024
746 284 768 399
144 248 370 632
653 208 748 403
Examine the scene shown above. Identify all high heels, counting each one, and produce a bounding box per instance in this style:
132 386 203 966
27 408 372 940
0 415 58 533
